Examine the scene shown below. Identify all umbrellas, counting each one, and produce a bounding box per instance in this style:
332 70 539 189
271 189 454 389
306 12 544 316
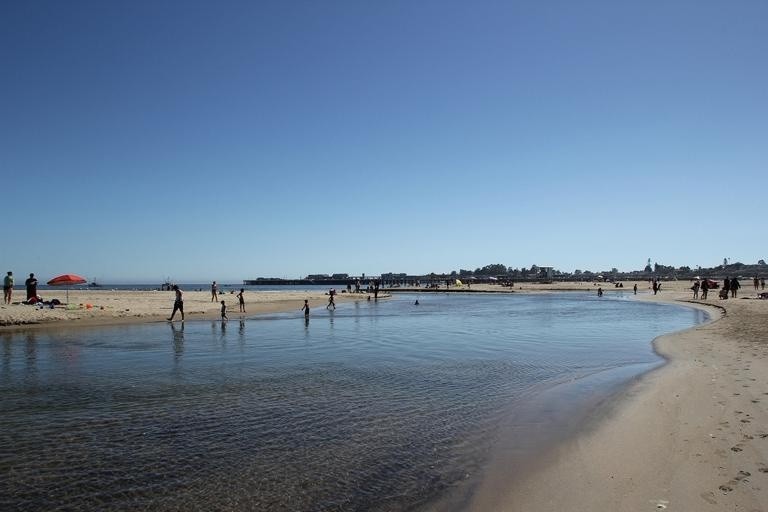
47 274 87 305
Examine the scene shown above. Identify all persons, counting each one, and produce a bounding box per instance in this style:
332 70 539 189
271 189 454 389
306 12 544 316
166 285 185 321
161 283 240 294
24 273 38 301
301 300 309 316
167 321 185 363
723 276 730 298
729 277 740 298
753 275 760 290
690 280 700 299
210 281 219 302
236 289 246 312
718 287 727 300
4 272 13 304
327 292 335 309
760 277 766 290
221 300 230 320
700 278 708 300
238 316 245 335
593 274 662 296
345 275 514 293
